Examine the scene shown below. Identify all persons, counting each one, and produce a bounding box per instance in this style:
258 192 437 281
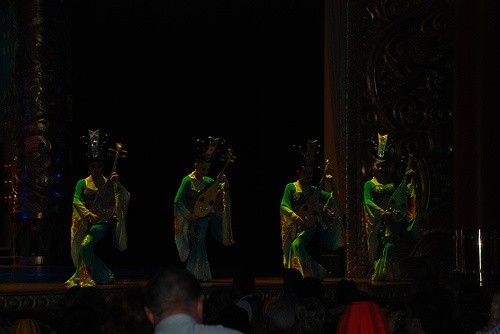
363 154 416 281
65 155 131 288
279 162 336 280
31 265 499 334
173 154 233 282
